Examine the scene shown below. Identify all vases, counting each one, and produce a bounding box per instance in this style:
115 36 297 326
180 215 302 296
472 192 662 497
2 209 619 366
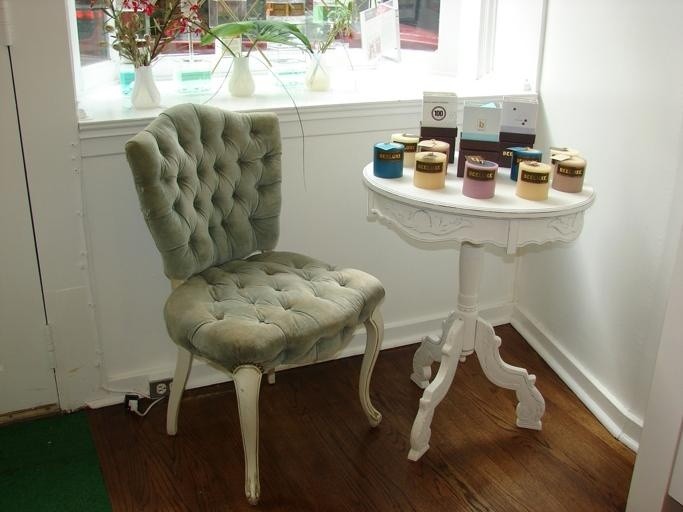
132 66 161 110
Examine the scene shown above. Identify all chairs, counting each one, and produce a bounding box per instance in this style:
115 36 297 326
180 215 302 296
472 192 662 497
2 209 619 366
124 102 386 507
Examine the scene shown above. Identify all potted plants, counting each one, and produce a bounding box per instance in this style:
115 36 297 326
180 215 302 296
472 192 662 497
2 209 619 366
194 0 379 191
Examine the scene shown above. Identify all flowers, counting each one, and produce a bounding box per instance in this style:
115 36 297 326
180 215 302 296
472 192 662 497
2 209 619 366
87 0 208 68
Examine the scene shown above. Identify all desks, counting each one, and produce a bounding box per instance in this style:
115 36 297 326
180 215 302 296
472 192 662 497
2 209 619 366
362 152 595 463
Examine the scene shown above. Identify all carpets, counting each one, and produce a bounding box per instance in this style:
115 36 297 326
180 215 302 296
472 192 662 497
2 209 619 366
1 410 114 512
